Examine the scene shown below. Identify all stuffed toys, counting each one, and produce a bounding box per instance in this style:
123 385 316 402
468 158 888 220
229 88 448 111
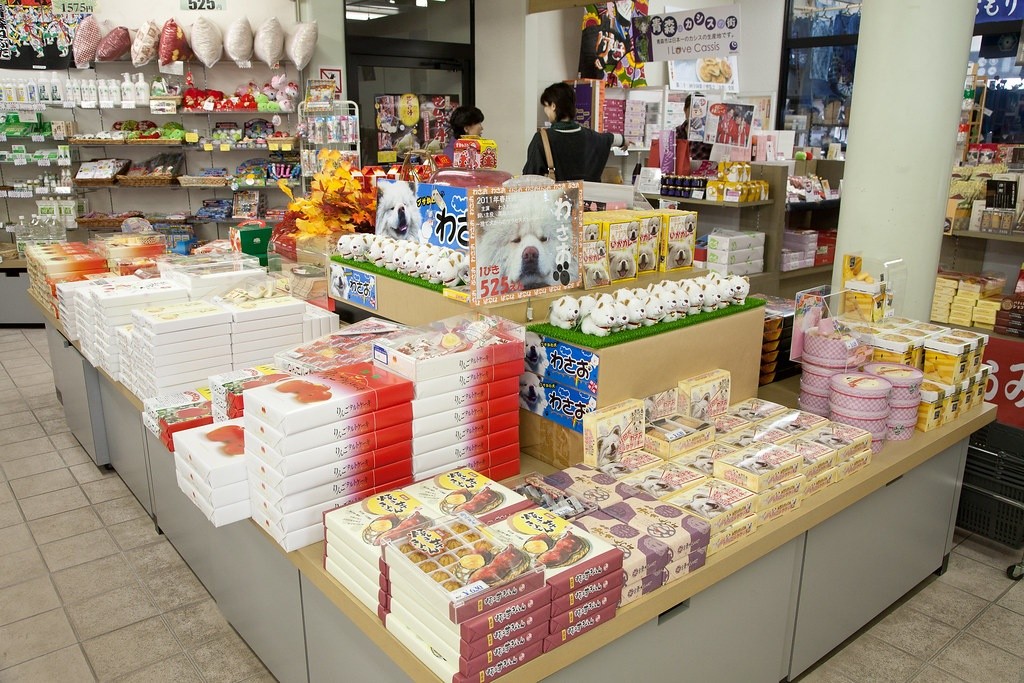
549 273 750 337
182 74 298 112
336 233 471 288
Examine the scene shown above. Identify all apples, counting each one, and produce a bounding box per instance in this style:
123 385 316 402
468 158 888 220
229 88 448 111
208 425 245 455
276 379 332 403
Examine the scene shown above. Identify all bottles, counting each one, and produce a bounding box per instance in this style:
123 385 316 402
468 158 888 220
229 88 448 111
0 71 150 103
14 166 74 190
16 214 67 261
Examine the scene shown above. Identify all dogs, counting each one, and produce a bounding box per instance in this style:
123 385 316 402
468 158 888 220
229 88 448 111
638 240 655 271
519 331 550 417
608 247 636 279
583 261 609 288
597 393 854 520
595 240 606 255
329 264 350 300
667 239 692 269
376 180 422 242
476 189 578 297
685 214 696 232
627 221 640 241
585 224 599 240
648 217 660 235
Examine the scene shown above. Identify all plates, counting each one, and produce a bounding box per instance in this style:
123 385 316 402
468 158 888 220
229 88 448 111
696 57 734 84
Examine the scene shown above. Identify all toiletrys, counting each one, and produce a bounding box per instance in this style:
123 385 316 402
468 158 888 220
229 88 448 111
0 71 150 102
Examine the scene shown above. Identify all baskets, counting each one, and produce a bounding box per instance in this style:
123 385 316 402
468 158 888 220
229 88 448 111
68 136 126 145
124 139 184 145
70 158 132 187
176 177 228 187
116 173 181 187
74 210 143 232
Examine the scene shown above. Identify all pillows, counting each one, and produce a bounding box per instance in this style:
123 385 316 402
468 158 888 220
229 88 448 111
285 20 317 70
253 18 284 69
131 21 162 67
191 13 224 68
72 13 102 69
222 15 253 68
159 18 196 65
94 27 131 63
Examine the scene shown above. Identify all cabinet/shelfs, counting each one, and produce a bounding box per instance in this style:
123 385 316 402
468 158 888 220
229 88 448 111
638 159 844 299
562 78 727 186
0 58 307 326
930 229 1024 429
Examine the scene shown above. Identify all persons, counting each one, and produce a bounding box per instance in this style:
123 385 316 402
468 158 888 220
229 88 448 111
717 108 749 144
523 83 636 183
443 106 484 163
675 92 713 160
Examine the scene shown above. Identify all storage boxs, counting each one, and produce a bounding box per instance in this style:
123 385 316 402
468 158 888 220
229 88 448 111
349 165 433 191
603 97 662 148
453 135 498 169
23 208 872 683
834 251 1024 454
